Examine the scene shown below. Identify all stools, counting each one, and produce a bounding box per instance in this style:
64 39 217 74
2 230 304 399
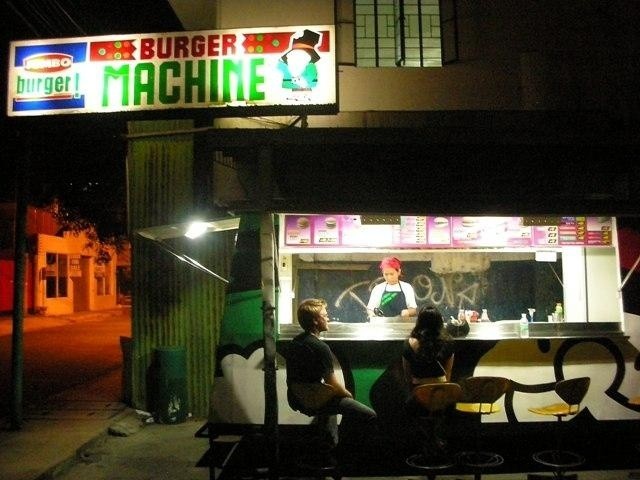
525 377 591 478
291 379 343 478
400 383 461 478
452 378 511 479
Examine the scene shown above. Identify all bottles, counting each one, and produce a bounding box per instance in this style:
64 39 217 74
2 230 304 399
520 313 529 338
554 303 562 323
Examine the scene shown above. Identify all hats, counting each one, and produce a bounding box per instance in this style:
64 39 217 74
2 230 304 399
380 256 402 271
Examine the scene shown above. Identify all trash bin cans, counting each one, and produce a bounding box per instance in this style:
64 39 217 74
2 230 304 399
148 346 189 424
119 336 132 405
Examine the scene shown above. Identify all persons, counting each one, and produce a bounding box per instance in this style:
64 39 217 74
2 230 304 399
366 256 419 320
400 304 457 413
285 298 378 465
